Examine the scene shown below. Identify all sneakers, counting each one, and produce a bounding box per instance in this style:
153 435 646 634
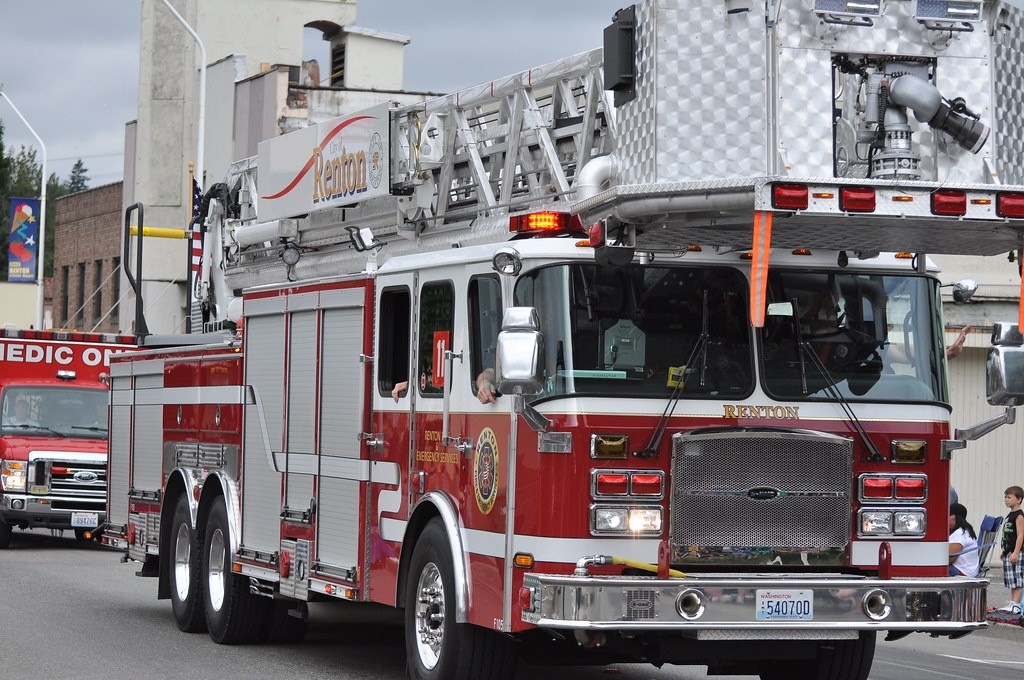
999 601 1023 614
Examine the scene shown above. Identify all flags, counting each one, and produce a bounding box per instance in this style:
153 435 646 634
192 175 212 280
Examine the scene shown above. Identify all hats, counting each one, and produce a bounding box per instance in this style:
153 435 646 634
792 304 854 343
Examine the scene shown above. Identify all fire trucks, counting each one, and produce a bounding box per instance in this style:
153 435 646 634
0 323 149 549
104 0 1024 680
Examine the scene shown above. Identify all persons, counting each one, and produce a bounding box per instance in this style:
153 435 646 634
951 486 958 503
805 284 972 377
392 381 407 403
997 486 1024 614
948 503 979 576
476 295 586 404
2 399 41 430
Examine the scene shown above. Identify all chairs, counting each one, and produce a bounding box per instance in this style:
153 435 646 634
584 263 657 334
679 270 793 345
